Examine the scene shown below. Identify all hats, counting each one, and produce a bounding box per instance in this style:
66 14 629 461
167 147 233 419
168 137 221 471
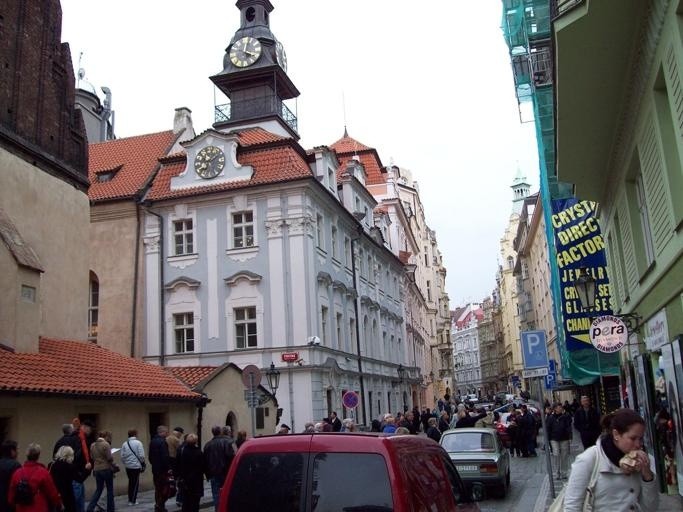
174 427 184 434
282 424 290 430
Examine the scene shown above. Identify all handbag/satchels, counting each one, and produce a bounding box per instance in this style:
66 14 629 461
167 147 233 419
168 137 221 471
547 445 599 512
163 479 177 497
141 462 146 472
72 480 83 500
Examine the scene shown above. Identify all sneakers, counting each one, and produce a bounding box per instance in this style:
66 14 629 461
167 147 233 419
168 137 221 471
555 477 567 482
511 452 537 458
154 507 168 512
129 502 139 506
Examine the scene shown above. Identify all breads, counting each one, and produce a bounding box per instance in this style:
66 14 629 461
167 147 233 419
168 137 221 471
620 450 649 475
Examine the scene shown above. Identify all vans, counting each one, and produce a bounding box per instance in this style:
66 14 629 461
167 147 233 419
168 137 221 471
218 433 480 512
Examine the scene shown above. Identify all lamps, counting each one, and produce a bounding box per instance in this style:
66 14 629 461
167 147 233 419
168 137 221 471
572 264 642 352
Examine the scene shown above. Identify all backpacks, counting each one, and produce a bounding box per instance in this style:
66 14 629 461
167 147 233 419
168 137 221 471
14 480 33 506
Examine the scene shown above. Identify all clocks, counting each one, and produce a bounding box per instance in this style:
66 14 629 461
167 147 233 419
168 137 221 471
273 42 289 74
229 37 262 69
193 144 226 180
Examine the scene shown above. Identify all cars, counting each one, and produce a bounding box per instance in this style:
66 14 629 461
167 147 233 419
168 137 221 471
621 394 675 458
436 427 512 497
481 390 541 447
458 394 478 404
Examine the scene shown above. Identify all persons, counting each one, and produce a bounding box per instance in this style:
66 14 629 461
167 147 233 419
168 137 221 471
0 438 21 512
562 408 660 511
47 417 248 511
668 379 683 495
275 384 604 482
6 442 65 512
